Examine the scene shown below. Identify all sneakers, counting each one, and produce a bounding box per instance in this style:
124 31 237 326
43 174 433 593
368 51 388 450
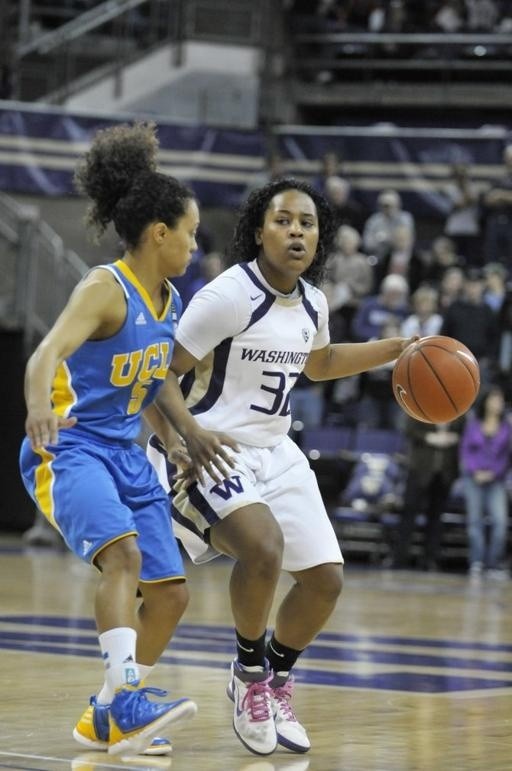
226 655 311 755
72 677 197 756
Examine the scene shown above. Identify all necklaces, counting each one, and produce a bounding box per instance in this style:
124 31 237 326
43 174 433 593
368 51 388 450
142 177 420 761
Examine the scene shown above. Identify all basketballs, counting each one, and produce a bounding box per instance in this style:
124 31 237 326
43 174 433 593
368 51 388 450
392 335 480 424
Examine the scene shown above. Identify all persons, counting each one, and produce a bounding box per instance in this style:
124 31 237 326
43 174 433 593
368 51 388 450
71 752 173 770
442 583 505 760
240 758 312 771
180 1 512 583
20 119 208 761
